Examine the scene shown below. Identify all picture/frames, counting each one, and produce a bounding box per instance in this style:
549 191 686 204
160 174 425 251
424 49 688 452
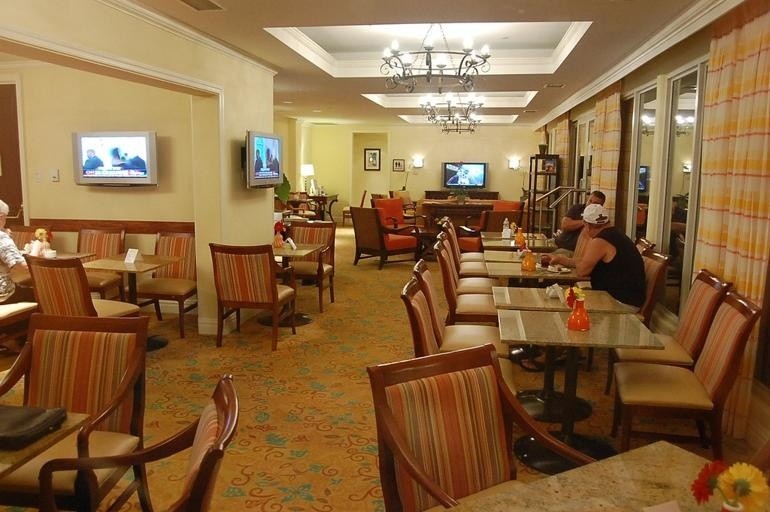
363 148 381 171
392 159 406 173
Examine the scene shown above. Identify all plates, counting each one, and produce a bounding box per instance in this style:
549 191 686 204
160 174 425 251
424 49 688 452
549 266 571 274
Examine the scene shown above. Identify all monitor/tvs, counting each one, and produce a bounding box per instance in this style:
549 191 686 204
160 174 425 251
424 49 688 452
71 130 158 187
245 129 284 190
443 162 487 190
638 166 647 192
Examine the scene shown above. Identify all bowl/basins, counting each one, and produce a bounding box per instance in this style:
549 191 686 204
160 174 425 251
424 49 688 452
23 241 57 259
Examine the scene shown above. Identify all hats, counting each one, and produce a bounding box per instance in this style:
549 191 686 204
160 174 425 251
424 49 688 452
581 204 609 225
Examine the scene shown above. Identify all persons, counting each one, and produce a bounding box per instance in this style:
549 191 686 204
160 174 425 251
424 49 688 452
82 149 104 172
0 200 35 353
510 204 647 370
547 191 606 259
254 147 279 175
446 169 478 185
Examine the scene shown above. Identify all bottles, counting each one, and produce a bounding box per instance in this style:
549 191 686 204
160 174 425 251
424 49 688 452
502 217 510 238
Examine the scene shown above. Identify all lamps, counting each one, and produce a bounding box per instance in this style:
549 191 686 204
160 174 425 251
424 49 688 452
380 24 494 135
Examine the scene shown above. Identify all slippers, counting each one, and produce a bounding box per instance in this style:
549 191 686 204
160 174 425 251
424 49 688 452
521 358 545 371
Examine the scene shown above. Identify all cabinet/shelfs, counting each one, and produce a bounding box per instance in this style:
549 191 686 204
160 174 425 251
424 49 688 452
527 154 559 235
